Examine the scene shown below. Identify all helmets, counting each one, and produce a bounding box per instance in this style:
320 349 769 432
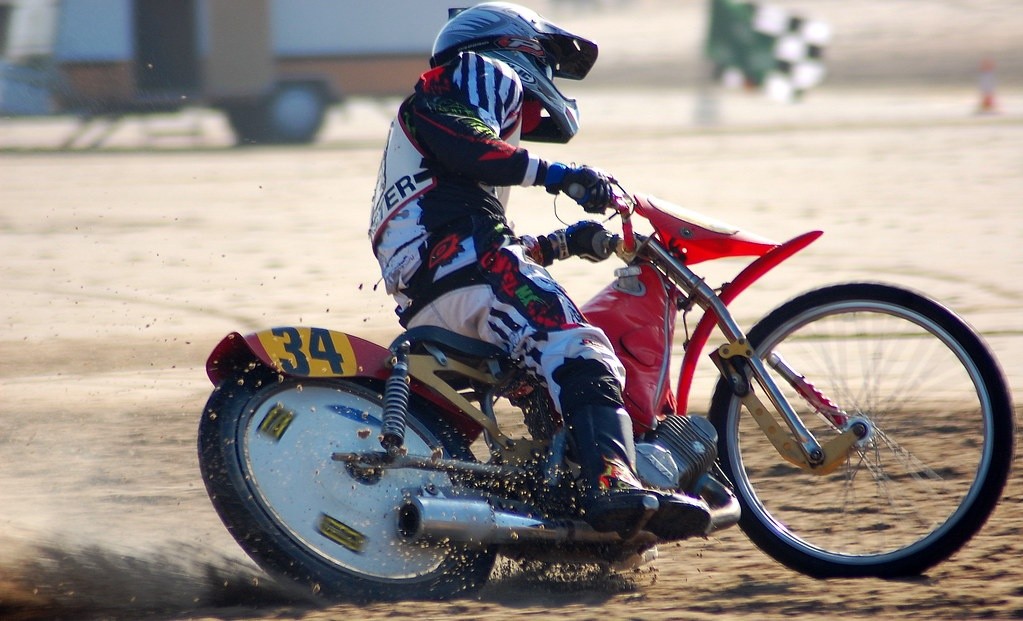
431 2 598 143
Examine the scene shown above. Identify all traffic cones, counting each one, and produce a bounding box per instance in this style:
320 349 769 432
969 49 1006 115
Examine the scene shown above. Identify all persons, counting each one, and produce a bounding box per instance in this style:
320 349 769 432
368 1 711 538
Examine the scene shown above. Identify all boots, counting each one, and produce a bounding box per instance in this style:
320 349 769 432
558 360 711 539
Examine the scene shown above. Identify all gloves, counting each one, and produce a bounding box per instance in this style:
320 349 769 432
548 221 606 262
543 163 611 214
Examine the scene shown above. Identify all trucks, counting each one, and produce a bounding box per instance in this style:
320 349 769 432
0 0 475 149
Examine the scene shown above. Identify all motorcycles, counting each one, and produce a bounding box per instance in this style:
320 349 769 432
197 182 1014 607
